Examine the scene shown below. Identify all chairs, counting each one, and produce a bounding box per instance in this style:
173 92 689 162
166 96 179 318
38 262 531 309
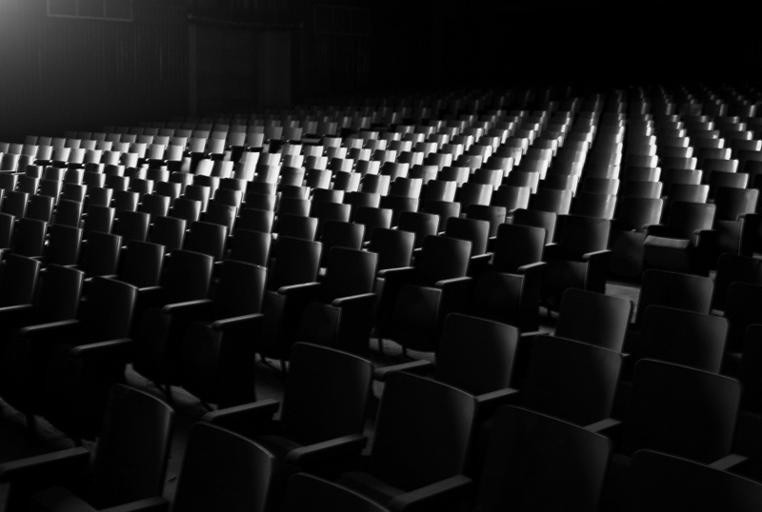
0 92 761 512
